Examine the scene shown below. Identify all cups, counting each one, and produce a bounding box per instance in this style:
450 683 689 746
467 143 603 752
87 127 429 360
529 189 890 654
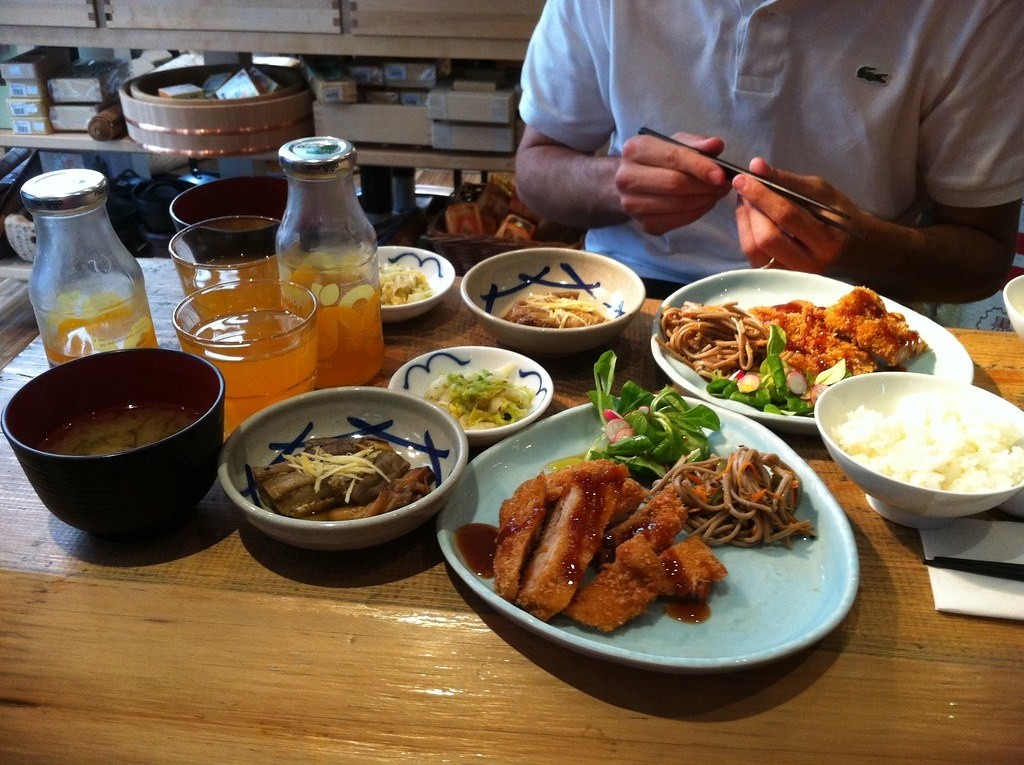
169 216 281 297
173 279 319 436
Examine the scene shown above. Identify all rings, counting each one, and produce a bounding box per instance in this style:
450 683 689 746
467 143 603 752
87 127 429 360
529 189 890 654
760 257 775 268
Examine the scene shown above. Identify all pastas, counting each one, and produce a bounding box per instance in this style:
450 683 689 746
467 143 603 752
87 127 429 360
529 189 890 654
651 445 818 551
653 299 769 378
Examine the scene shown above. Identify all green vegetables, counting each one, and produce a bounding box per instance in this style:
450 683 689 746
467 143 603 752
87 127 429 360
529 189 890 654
705 325 852 418
583 350 721 481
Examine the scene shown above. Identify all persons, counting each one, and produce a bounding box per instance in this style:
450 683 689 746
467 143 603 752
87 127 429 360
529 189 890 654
516 1 1024 311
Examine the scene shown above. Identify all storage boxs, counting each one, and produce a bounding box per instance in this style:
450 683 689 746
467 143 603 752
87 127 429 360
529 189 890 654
0 45 525 157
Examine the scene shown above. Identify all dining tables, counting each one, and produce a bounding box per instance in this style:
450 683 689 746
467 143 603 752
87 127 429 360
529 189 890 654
0 255 1024 765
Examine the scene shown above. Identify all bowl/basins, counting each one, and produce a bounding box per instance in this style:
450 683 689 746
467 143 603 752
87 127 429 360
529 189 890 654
388 345 554 448
378 246 457 324
1003 274 1024 342
169 175 289 264
461 246 647 355
0 348 226 534
814 372 1024 530
217 386 468 553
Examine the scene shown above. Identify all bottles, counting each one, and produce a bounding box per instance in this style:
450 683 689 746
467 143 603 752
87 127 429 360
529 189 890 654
274 136 385 390
20 167 160 372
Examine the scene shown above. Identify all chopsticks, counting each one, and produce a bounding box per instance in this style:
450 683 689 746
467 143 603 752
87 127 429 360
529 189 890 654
638 127 870 240
923 556 1024 582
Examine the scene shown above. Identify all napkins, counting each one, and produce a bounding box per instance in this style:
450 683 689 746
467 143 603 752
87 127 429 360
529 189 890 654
915 516 1024 621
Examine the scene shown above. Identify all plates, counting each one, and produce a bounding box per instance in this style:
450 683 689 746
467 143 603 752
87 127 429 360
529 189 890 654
651 269 974 436
436 395 860 676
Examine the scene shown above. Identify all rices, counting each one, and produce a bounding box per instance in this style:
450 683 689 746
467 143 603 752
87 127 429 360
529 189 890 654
832 385 1024 492
